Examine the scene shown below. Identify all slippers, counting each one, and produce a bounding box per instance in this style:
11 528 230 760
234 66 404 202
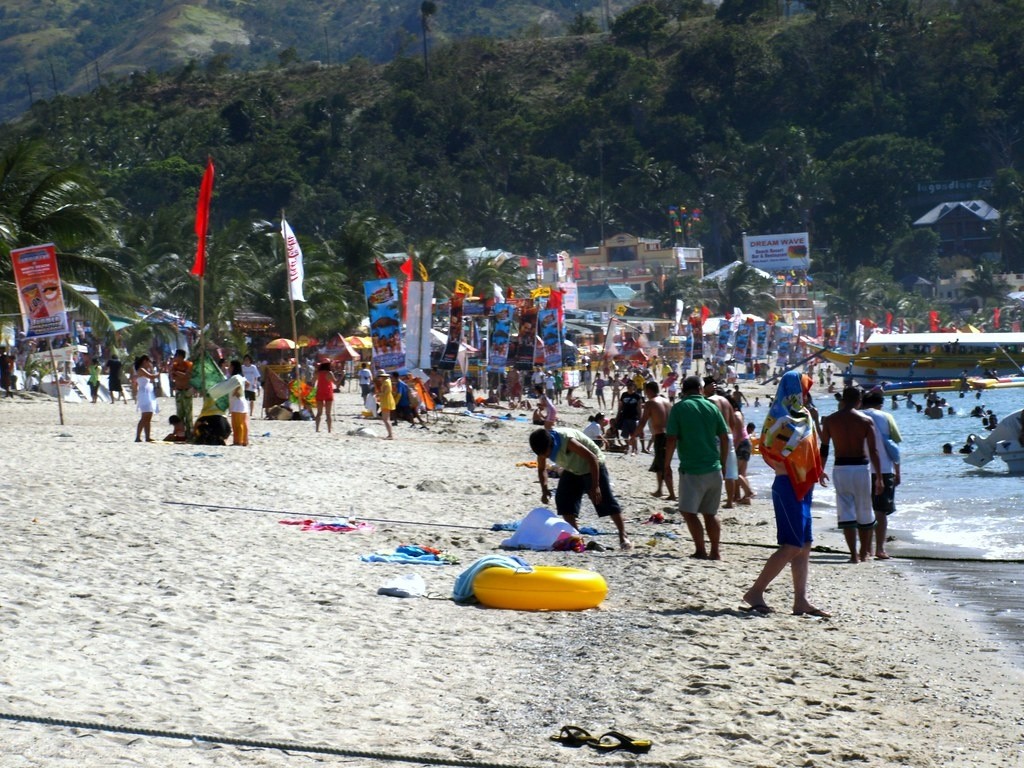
586 731 652 753
550 726 598 743
792 609 832 618
739 605 774 618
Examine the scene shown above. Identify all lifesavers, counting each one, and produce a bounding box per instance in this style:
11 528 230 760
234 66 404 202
473 567 607 610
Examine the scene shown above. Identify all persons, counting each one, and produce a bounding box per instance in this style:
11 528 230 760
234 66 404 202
357 363 427 440
0 347 17 394
879 368 999 453
466 356 833 509
818 387 904 562
130 354 260 446
663 377 729 558
739 372 833 617
280 353 346 433
530 428 632 550
430 369 452 399
88 355 127 405
518 319 535 346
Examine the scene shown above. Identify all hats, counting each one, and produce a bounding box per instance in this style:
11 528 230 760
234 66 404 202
375 368 391 377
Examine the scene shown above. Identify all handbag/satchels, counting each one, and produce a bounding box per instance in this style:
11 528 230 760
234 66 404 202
613 411 623 429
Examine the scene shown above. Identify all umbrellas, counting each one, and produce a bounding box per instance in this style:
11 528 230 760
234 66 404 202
345 335 373 375
266 338 301 357
298 335 319 355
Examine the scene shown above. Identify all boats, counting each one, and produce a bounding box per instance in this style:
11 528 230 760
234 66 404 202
803 332 1024 387
